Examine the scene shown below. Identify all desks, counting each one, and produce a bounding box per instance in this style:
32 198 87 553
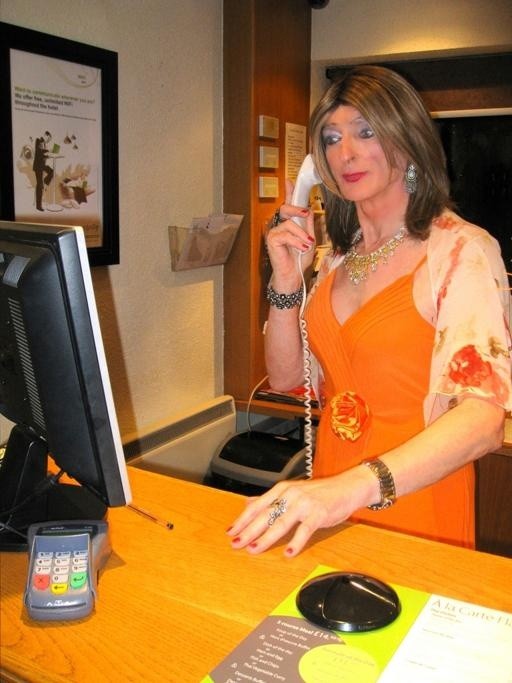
1 456 512 683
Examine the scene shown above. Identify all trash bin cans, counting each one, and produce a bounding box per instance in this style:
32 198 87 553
202 430 314 497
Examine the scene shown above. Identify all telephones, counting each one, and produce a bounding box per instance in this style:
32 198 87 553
290 153 322 253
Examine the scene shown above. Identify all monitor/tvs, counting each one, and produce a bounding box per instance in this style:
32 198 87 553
0 220 134 552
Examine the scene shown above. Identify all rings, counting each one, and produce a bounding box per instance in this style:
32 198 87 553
268 498 289 526
274 209 289 225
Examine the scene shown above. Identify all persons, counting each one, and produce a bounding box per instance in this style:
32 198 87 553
225 60 511 556
29 131 57 212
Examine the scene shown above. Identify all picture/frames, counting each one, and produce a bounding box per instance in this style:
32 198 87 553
0 20 120 265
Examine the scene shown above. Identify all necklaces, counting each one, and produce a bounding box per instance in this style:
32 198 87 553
342 223 415 285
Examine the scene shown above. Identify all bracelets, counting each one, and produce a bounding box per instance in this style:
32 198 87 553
361 458 396 512
265 285 304 310
